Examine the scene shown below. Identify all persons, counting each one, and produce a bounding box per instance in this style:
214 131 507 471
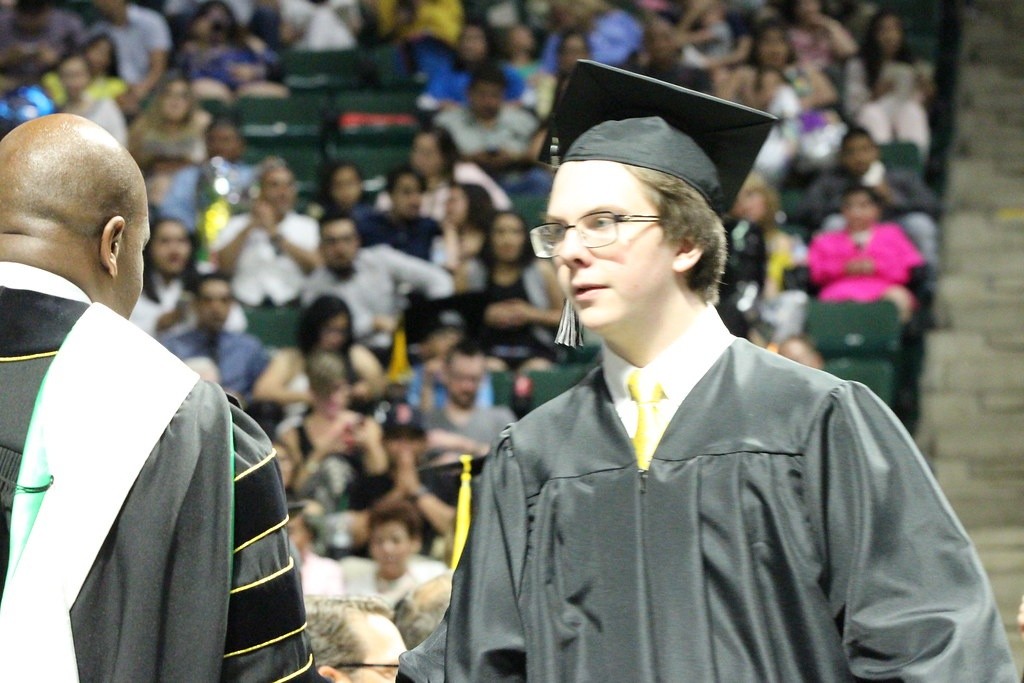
0 0 940 683
0 112 322 682
395 60 1020 682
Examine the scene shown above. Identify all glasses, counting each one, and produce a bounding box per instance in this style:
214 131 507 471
528 210 667 259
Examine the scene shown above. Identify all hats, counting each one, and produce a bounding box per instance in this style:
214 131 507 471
536 58 779 351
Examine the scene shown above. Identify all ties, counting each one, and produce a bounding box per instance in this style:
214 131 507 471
627 370 666 473
